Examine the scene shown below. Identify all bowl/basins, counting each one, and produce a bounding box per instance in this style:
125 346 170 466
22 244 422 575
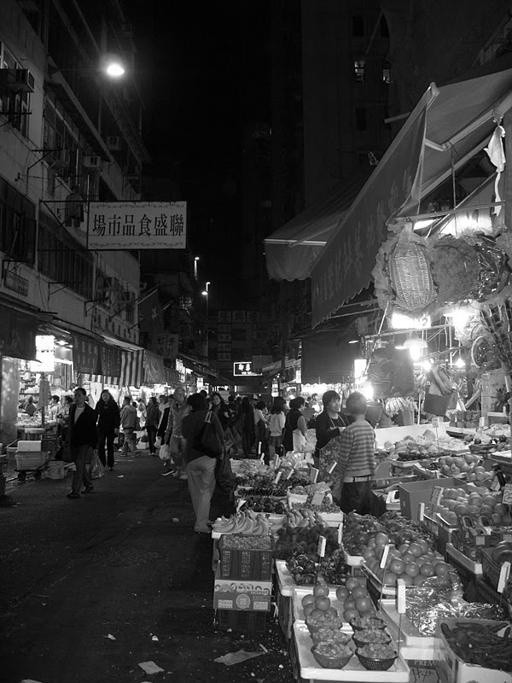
303 615 399 671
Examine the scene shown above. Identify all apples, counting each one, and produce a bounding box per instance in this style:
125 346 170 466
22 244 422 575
302 531 451 623
432 454 512 524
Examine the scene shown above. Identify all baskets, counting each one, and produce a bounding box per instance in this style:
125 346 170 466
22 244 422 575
349 616 398 670
305 618 354 668
220 534 277 581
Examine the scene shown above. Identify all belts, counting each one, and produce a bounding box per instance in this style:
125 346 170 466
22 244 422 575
343 476 371 483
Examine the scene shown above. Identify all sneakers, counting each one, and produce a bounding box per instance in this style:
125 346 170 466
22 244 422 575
194 519 214 534
66 483 93 498
161 468 174 476
172 471 188 480
119 453 141 458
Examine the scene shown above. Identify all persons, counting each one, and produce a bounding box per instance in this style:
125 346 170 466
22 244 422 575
22 387 376 535
426 368 451 423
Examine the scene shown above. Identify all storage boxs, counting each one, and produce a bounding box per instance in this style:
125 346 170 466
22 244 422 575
433 618 512 683
214 562 272 614
397 477 468 522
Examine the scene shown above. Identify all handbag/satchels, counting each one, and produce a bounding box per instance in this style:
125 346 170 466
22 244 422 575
193 420 222 458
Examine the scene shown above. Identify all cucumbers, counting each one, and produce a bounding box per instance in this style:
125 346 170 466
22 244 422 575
438 618 511 672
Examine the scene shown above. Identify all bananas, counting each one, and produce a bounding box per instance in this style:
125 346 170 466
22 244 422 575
208 507 328 539
